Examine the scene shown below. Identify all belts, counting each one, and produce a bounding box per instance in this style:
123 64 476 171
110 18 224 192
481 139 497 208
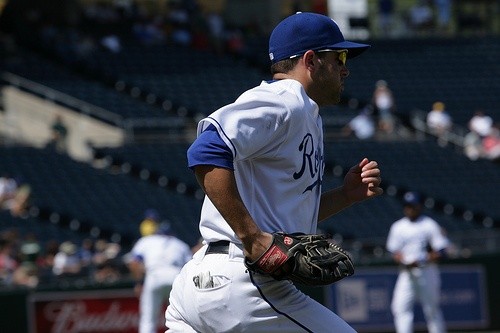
205 240 230 254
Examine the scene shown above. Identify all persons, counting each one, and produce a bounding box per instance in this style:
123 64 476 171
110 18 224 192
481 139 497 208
163 10 384 333
0 0 500 333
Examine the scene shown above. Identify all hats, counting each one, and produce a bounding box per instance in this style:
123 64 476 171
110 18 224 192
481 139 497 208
140 220 158 236
402 191 419 205
269 12 372 63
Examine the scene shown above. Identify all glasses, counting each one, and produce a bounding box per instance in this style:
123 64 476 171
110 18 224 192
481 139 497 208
290 51 347 66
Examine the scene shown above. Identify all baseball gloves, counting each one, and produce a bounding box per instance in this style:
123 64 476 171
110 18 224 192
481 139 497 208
244 230 355 286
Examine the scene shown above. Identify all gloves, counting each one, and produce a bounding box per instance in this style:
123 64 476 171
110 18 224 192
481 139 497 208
404 253 427 268
